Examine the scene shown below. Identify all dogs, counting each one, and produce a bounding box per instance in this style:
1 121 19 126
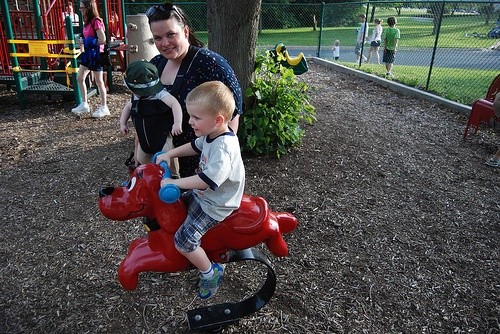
97 157 299 292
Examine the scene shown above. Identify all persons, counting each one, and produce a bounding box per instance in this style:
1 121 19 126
330 39 341 62
119 60 184 179
60 1 81 27
155 80 247 301
129 3 243 224
71 0 114 118
354 12 369 64
365 17 383 65
381 15 401 77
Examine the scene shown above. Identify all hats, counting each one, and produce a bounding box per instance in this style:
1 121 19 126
122 59 165 96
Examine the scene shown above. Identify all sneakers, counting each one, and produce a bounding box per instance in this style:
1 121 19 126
198 262 225 300
92 105 110 117
71 103 90 113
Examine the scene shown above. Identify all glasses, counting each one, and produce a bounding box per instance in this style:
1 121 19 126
146 3 184 18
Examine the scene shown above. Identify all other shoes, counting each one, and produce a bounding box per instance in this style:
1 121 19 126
485 157 500 167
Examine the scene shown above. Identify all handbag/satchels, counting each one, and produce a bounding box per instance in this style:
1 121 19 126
99 44 112 67
370 39 380 46
